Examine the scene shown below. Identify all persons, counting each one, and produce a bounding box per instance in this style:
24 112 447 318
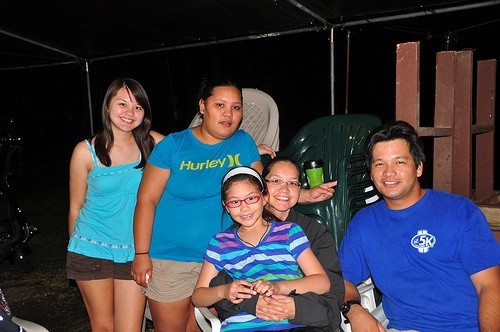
191 166 331 332
337 120 500 332
131 80 337 332
209 155 345 332
65 79 277 332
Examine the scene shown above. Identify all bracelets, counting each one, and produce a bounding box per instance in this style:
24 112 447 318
136 252 150 255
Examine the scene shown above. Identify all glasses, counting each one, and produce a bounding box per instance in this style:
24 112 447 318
224 188 265 208
264 178 301 189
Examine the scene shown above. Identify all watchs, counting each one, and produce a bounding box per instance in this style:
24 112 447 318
340 300 361 323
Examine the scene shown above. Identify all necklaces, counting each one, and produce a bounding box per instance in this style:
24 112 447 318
236 221 270 248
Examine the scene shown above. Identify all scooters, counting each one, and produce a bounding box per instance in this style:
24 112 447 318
0 115 38 265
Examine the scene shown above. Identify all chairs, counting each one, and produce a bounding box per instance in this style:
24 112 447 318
189 88 389 332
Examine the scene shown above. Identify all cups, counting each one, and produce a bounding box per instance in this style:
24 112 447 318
305 160 326 189
